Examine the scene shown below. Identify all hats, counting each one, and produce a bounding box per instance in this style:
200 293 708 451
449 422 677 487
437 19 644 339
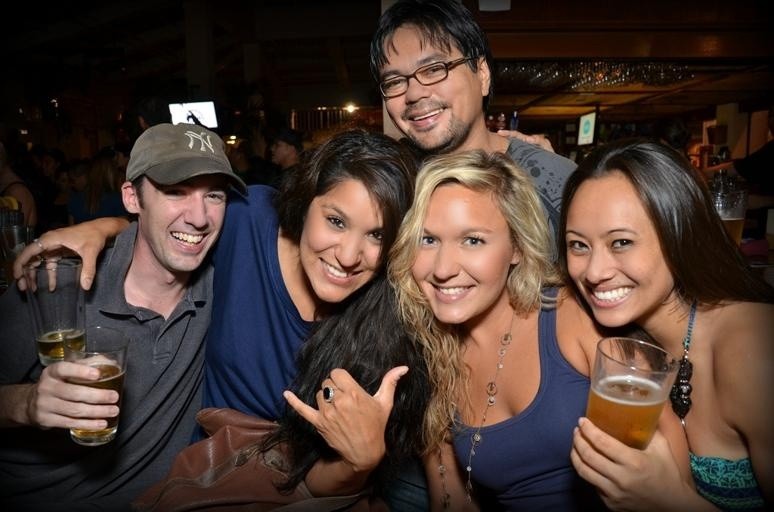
124 122 249 197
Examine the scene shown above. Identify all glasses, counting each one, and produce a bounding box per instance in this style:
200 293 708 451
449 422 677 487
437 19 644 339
378 52 482 100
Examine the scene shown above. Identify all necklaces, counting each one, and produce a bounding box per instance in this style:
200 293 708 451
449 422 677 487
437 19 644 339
659 299 699 418
438 312 516 509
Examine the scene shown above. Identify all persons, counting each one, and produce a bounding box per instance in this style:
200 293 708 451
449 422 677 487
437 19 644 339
0 72 305 226
364 1 577 267
0 121 411 509
388 151 689 512
702 121 774 198
496 128 774 511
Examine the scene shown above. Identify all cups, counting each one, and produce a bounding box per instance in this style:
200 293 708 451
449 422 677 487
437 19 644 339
2 225 34 282
584 338 678 452
712 186 746 246
25 262 91 370
62 332 128 445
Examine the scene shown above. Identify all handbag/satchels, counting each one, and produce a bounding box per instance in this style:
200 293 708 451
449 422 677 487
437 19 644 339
123 403 401 511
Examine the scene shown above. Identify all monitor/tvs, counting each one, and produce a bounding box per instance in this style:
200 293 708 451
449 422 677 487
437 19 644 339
168 100 221 132
574 111 598 148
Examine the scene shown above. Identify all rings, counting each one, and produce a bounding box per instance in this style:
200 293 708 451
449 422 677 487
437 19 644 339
324 385 336 401
